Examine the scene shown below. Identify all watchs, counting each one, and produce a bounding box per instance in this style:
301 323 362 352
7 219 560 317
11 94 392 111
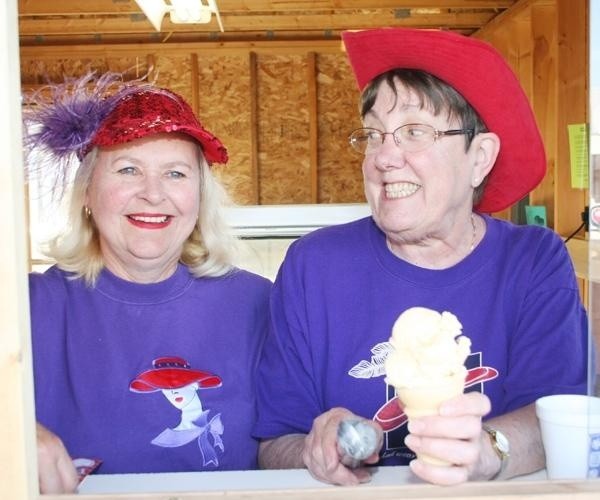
482 422 510 481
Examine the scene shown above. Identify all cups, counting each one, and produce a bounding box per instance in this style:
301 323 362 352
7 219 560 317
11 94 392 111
387 372 469 470
536 395 600 481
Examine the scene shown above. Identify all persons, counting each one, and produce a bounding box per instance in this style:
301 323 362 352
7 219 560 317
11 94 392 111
30 85 273 496
253 28 595 486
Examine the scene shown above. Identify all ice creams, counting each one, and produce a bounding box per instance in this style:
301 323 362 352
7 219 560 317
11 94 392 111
385 306 472 470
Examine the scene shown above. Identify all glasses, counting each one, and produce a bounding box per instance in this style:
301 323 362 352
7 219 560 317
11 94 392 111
348 123 474 154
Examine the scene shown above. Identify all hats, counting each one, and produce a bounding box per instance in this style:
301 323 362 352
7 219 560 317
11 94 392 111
22 65 229 167
341 28 547 213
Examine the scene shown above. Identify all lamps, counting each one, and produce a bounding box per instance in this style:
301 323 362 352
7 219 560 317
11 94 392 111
136 0 226 35
